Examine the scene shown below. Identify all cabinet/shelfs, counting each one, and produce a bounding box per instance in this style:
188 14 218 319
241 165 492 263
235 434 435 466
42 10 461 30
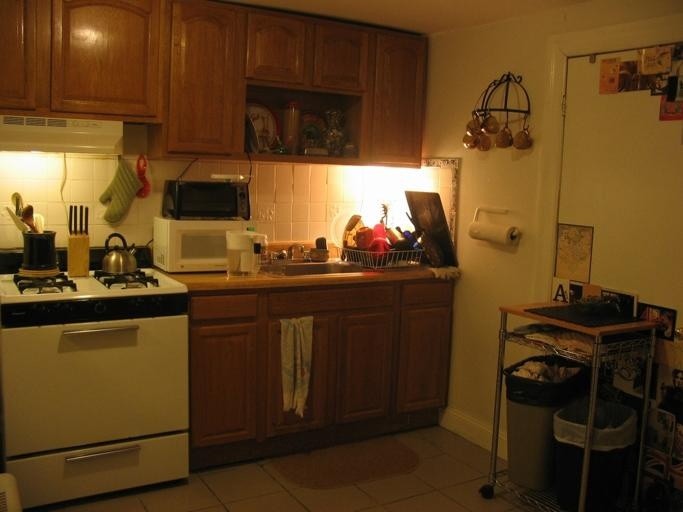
144 1 245 161
340 28 429 170
245 6 371 165
0 1 172 126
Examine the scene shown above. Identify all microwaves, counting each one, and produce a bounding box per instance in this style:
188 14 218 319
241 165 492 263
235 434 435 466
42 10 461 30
153 218 252 273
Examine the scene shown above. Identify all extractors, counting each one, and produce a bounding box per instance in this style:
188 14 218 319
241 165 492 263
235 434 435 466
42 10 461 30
0 115 125 154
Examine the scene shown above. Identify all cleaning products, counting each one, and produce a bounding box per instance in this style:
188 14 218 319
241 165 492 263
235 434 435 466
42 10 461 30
254 235 268 265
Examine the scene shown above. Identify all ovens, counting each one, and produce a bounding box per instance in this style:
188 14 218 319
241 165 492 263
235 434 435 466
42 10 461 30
0 315 190 508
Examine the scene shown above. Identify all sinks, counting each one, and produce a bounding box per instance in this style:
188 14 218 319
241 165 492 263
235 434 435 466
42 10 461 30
262 261 357 280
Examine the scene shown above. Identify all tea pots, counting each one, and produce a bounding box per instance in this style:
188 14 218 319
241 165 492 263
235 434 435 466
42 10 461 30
102 232 137 272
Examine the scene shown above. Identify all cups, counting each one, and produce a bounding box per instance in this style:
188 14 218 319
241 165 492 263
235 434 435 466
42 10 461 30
461 115 533 153
225 228 267 280
22 230 56 270
354 224 409 257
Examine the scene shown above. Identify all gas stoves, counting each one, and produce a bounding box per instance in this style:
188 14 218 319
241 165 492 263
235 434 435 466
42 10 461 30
0 267 188 324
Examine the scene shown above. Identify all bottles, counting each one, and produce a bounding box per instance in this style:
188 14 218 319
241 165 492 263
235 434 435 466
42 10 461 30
284 98 355 158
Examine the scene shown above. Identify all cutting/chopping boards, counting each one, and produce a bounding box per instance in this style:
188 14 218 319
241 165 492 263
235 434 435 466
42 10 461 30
403 189 459 268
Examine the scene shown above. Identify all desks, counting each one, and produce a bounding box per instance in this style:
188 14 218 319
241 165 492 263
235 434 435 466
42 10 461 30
478 300 658 511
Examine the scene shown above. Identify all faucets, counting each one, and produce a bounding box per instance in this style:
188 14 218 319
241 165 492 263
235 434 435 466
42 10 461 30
288 243 304 258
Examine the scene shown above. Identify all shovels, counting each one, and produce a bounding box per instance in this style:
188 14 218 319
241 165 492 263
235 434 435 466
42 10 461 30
341 215 361 261
23 206 39 234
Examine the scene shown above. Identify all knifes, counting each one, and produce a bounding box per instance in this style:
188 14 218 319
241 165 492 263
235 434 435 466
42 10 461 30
67 204 89 234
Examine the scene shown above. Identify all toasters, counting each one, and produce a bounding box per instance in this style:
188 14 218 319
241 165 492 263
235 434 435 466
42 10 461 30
163 177 254 219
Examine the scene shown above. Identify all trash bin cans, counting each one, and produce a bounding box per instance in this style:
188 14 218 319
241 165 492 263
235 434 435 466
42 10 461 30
503 355 591 492
553 400 637 512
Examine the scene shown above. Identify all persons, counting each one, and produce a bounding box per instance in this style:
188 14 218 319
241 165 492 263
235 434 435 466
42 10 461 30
661 310 672 338
660 369 683 424
672 420 683 460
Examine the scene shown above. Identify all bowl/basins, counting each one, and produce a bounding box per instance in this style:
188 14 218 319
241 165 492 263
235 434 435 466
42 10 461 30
246 97 279 152
